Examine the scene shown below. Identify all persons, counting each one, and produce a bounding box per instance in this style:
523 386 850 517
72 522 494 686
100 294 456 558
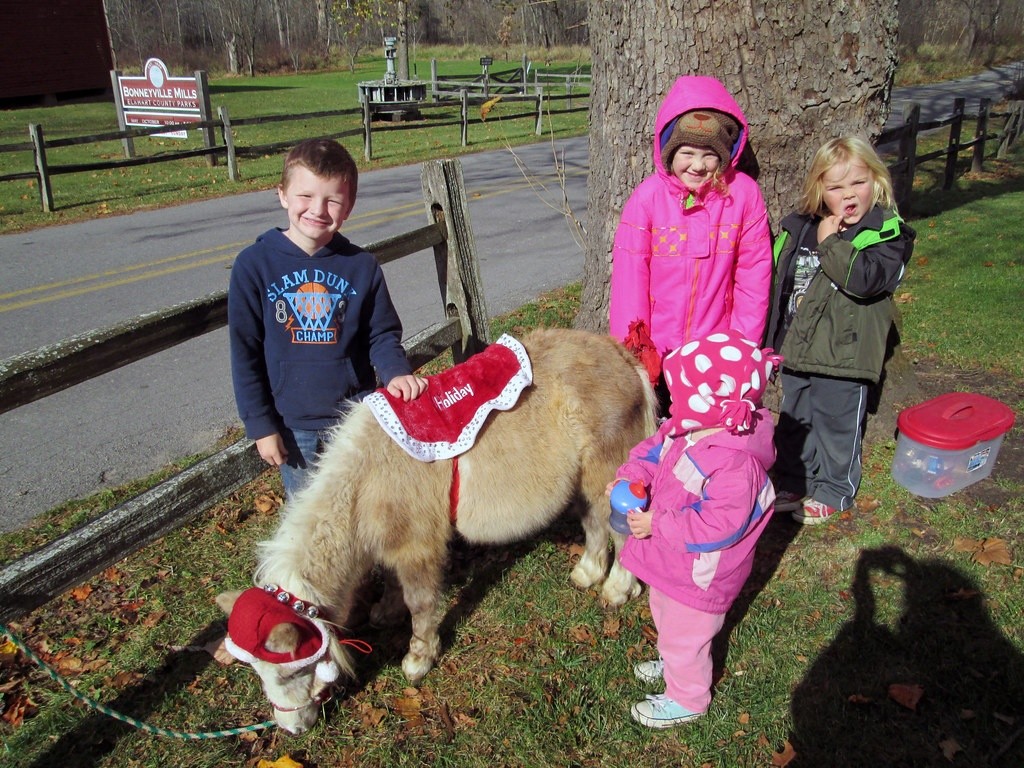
607 76 773 419
604 331 785 729
761 137 916 523
227 139 429 502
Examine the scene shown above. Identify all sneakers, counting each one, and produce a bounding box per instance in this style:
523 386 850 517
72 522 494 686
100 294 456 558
773 489 807 511
792 497 839 525
630 690 710 728
634 659 665 682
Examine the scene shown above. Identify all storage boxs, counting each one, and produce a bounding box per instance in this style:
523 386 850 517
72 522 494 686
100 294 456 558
894 395 1014 499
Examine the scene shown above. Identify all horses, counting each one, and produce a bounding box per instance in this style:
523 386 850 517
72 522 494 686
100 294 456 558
216 327 660 739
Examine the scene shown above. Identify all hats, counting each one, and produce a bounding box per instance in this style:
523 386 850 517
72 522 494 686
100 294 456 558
660 111 738 175
663 328 785 438
